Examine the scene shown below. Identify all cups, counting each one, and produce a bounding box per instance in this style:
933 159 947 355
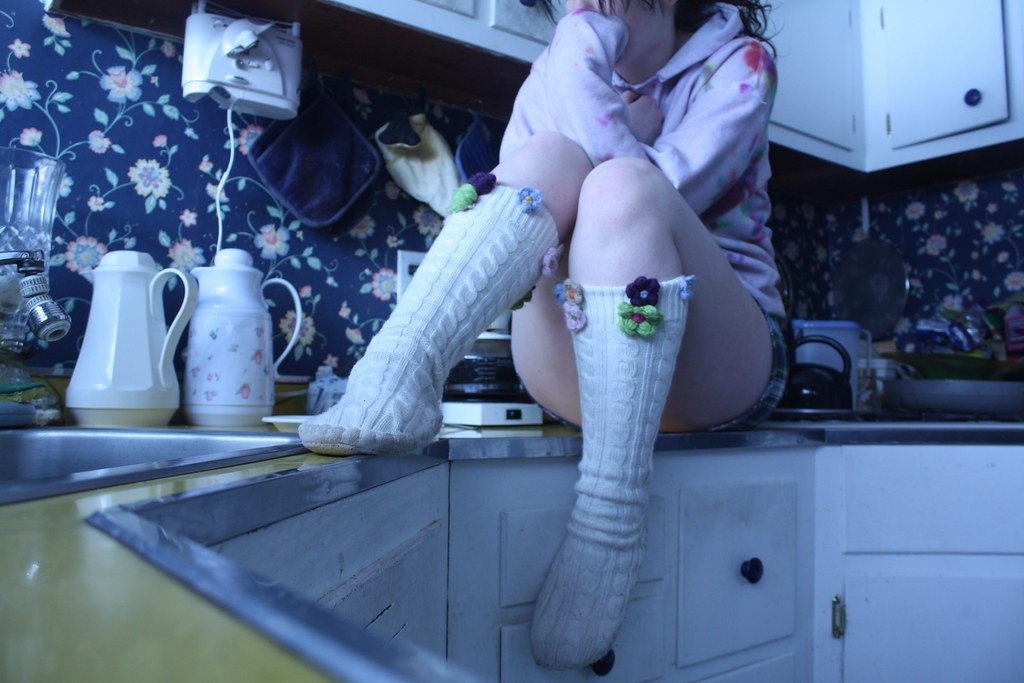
0 144 66 305
855 356 896 417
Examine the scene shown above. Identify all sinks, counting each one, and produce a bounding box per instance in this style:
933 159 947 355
1 398 310 507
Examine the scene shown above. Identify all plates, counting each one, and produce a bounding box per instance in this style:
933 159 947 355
886 376 1024 412
259 411 444 433
826 241 908 339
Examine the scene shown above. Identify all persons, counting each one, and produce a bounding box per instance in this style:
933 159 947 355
297 0 792 669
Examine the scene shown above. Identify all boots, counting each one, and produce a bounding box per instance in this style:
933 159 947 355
529 276 702 675
297 170 563 455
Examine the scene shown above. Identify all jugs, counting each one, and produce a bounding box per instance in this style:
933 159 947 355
791 320 872 411
783 336 852 408
184 250 303 425
64 249 200 425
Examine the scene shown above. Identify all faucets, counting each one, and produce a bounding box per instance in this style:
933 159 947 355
0 249 72 342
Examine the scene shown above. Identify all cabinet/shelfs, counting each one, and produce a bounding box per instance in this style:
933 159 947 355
42 0 1024 173
208 445 1024 683
